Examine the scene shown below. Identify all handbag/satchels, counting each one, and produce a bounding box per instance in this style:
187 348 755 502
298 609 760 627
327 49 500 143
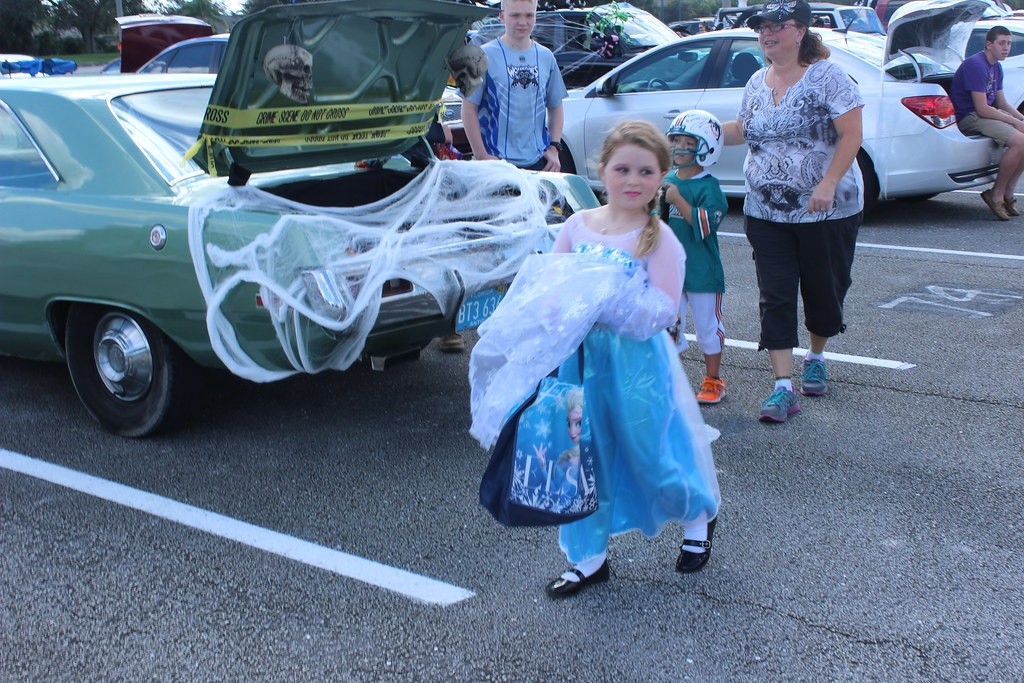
479 341 599 525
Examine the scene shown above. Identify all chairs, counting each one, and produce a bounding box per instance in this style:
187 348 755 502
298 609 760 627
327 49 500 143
727 49 763 90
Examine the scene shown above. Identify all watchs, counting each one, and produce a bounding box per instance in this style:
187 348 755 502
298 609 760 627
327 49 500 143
550 141 562 152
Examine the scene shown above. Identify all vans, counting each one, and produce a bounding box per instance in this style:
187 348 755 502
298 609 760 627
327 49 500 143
468 1 683 90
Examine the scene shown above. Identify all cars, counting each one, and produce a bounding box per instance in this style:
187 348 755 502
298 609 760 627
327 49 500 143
99 13 230 75
0 53 49 78
438 85 474 160
544 0 1024 218
1 2 602 439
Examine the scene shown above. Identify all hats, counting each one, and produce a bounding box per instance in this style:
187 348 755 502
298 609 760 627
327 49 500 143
746 0 811 29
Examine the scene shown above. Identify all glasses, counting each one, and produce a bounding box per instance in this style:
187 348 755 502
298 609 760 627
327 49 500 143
754 22 804 33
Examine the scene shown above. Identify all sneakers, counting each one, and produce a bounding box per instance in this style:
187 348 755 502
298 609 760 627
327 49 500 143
759 386 800 421
800 351 828 394
1004 196 1020 216
980 189 1010 220
697 375 726 403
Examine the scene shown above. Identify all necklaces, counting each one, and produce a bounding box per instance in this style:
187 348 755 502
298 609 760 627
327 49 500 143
600 224 622 232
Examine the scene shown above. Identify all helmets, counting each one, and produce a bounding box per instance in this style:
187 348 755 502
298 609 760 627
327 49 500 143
666 109 724 167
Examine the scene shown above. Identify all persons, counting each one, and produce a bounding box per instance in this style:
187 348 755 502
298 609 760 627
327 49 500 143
723 0 865 420
456 0 570 171
402 112 464 351
657 111 729 404
950 25 1024 220
544 122 721 598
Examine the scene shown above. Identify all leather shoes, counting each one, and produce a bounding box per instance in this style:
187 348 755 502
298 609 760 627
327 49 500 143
546 558 609 597
677 515 717 572
